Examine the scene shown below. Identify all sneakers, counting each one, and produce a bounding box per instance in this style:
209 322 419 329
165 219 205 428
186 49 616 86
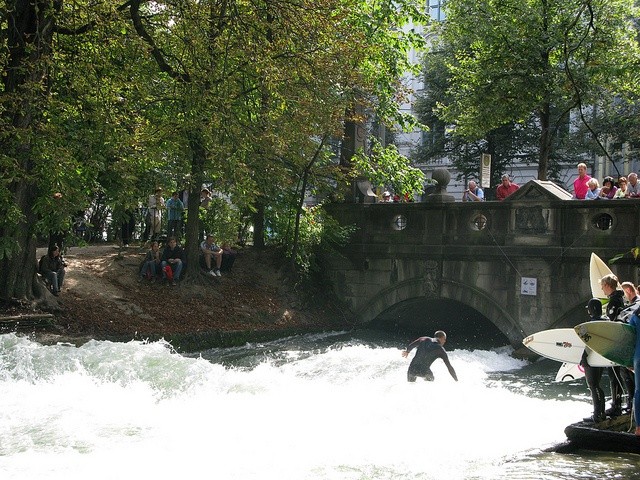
207 270 217 276
214 269 221 277
171 279 176 285
165 280 170 286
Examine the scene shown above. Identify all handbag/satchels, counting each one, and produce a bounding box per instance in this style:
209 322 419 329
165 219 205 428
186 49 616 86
164 266 173 281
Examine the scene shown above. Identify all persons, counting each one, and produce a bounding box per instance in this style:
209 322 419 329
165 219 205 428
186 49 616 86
463 181 484 201
495 174 520 201
166 192 184 244
39 246 65 296
579 298 608 424
199 235 224 277
377 191 394 203
613 177 628 199
627 173 640 199
137 241 163 285
148 189 165 240
585 178 602 200
573 163 592 199
199 189 213 243
121 203 135 248
620 280 640 438
161 238 184 287
600 275 624 417
600 176 617 200
402 330 458 382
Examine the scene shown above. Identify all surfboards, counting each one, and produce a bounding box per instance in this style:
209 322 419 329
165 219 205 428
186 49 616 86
590 252 623 298
586 350 623 367
574 321 634 369
552 362 585 383
522 328 586 364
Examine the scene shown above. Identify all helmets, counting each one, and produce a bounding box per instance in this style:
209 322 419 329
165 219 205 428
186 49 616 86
585 298 602 316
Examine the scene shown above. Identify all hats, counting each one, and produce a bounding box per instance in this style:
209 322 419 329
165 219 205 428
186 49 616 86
382 192 390 197
